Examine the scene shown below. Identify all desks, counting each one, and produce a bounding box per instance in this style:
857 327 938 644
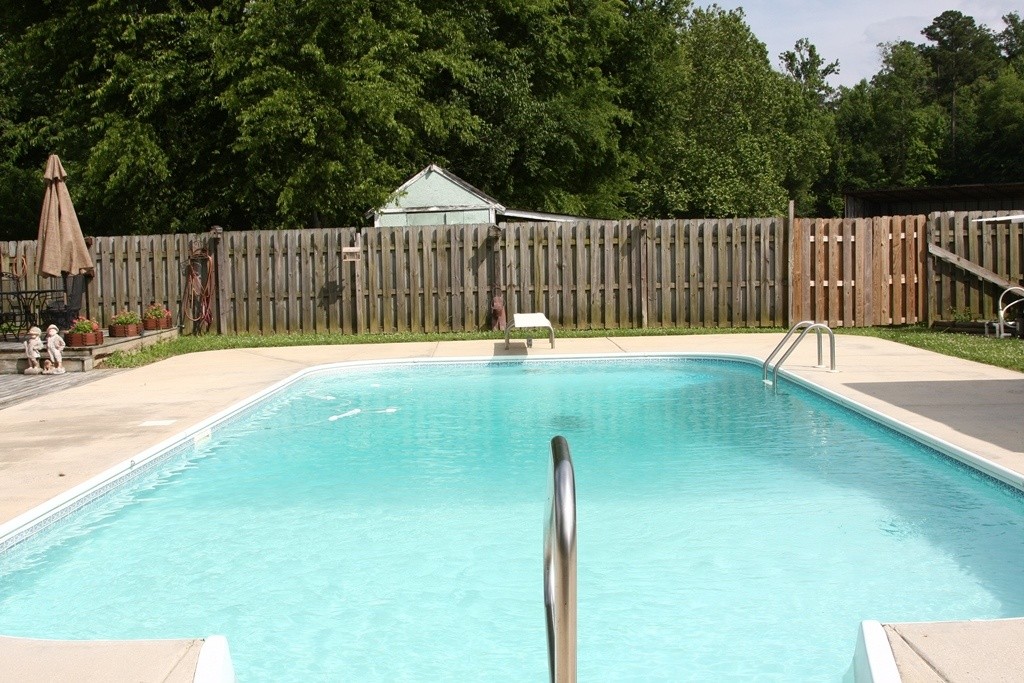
0 289 66 338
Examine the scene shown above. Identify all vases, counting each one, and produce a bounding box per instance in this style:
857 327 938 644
64 331 104 346
108 321 143 336
144 317 171 330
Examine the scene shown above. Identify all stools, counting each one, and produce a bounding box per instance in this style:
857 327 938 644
505 312 555 350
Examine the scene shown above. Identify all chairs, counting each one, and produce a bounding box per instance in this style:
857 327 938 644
0 272 20 342
50 275 86 323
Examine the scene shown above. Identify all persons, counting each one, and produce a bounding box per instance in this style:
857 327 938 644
25 324 66 368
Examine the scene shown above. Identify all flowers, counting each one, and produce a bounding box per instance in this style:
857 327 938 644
69 316 100 332
144 303 172 318
111 308 142 324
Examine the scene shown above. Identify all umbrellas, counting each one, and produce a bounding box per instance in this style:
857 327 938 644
34 154 96 279
